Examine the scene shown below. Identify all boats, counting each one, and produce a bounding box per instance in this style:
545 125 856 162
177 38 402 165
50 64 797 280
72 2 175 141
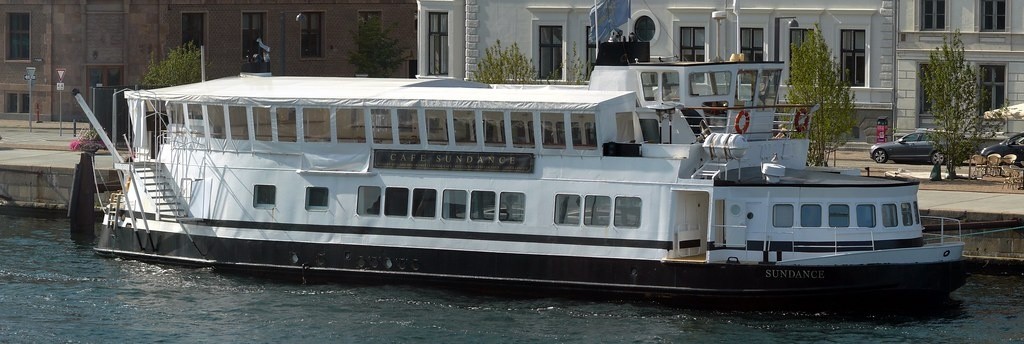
702 131 750 160
93 0 971 318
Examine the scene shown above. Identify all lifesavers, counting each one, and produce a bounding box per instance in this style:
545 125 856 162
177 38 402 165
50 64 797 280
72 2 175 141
795 108 808 131
734 110 751 134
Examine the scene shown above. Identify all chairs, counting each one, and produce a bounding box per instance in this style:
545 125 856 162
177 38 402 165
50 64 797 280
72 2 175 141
972 153 1024 190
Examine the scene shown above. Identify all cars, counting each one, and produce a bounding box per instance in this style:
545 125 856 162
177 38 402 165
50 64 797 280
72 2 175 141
980 132 1024 164
870 130 967 165
